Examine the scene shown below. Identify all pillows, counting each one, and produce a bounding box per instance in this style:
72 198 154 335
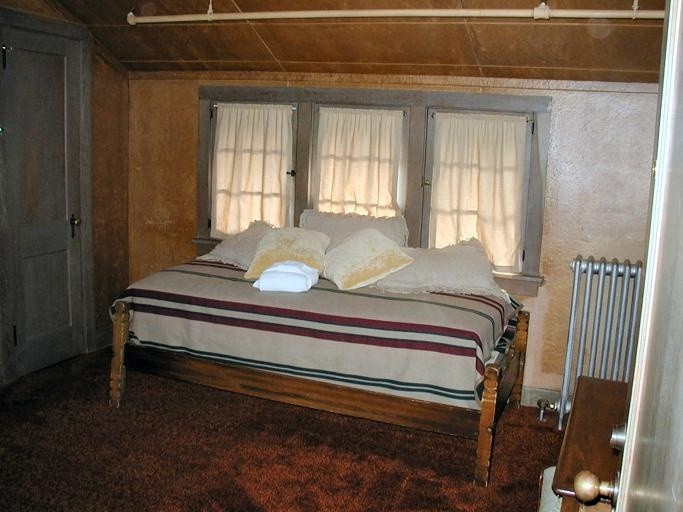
376 236 512 304
195 220 281 270
241 228 331 280
300 209 409 253
321 227 416 291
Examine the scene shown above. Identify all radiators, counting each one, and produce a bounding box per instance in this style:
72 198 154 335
537 254 643 431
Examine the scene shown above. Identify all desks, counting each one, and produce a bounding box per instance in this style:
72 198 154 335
551 375 633 512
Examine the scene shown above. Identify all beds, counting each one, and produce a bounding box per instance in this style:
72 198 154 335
108 256 531 487
